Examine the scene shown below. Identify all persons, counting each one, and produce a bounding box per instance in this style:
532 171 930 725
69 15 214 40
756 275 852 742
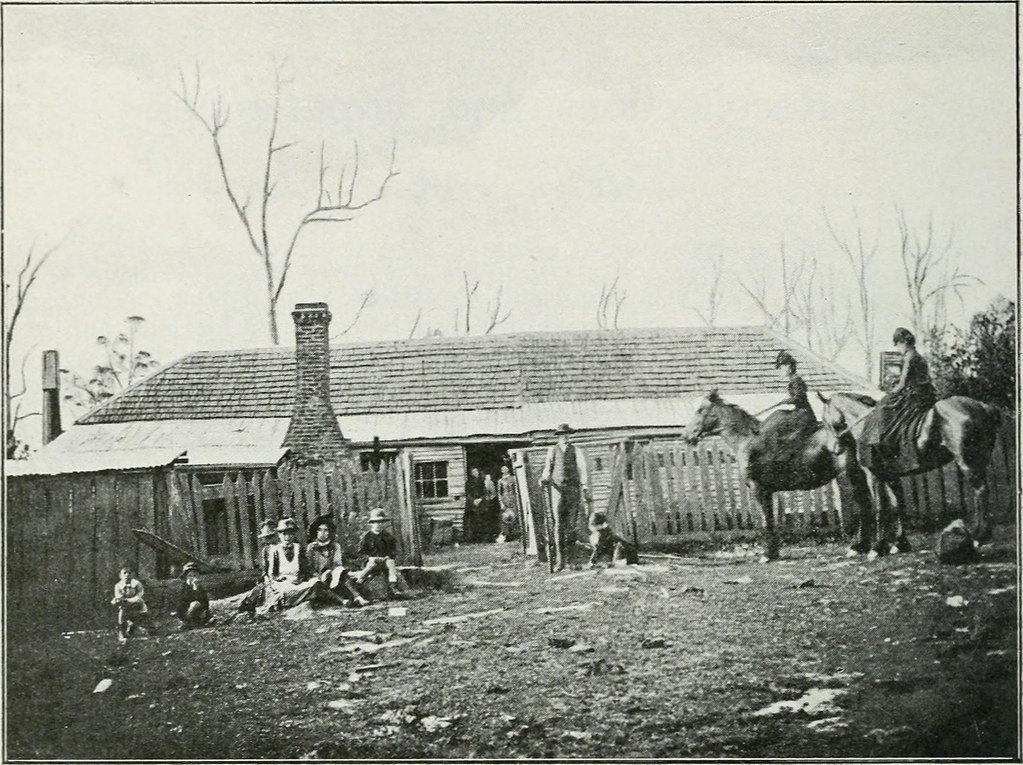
357 507 417 603
868 327 936 470
257 518 370 612
768 355 816 484
114 559 144 642
465 464 518 543
539 423 590 572
177 562 216 630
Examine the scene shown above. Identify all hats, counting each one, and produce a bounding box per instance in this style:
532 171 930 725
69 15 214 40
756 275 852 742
589 513 608 531
368 508 391 523
893 328 910 346
308 515 336 538
258 525 275 538
502 508 516 524
554 424 577 435
776 351 796 369
274 518 299 531
179 562 201 579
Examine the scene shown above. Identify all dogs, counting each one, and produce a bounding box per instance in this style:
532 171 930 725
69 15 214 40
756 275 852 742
587 512 647 568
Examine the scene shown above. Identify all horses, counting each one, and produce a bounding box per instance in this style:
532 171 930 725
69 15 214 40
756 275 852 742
679 385 873 557
816 389 1005 558
